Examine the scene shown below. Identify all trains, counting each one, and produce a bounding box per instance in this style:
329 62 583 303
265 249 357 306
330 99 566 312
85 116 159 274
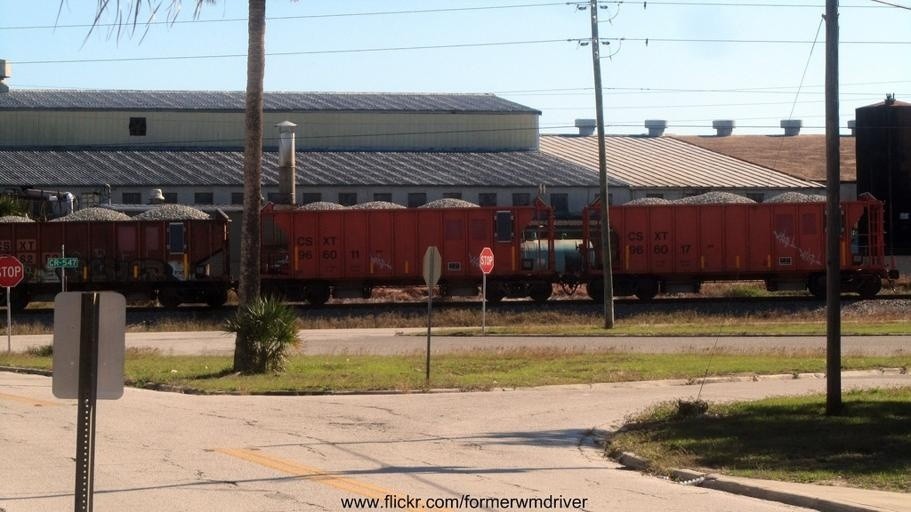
0 184 901 306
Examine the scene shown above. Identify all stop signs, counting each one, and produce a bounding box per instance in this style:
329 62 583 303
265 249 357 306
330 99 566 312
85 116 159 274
480 248 495 273
1 256 24 288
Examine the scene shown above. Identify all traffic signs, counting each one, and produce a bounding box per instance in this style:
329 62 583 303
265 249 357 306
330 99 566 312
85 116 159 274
49 258 78 267
